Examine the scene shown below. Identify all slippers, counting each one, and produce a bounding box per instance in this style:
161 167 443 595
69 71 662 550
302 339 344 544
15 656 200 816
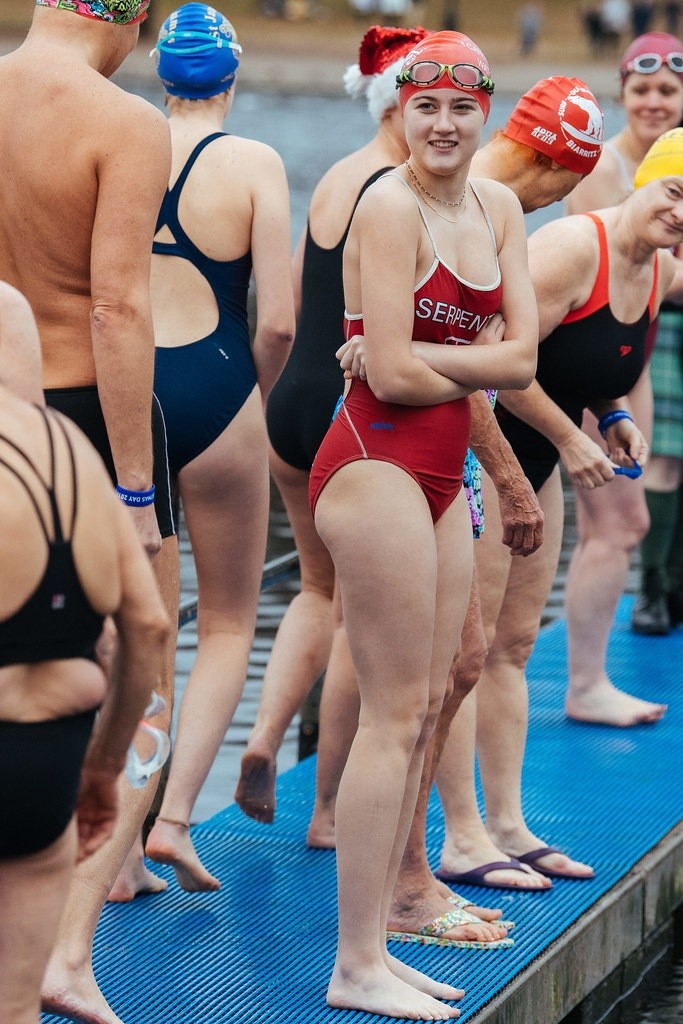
436 854 553 892
387 909 514 948
509 847 594 878
447 893 515 930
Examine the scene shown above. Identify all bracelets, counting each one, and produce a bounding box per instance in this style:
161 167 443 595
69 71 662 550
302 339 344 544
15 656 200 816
598 410 634 440
116 484 156 507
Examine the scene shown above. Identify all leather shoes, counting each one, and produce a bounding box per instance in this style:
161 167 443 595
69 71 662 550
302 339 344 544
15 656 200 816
631 579 672 635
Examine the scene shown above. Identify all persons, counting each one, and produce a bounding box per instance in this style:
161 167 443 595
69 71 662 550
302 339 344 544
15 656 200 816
0 0 683 1024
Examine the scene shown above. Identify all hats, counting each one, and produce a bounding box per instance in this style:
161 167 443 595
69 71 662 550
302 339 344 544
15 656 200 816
399 31 490 125
503 76 604 174
342 24 432 125
632 127 683 191
619 33 683 85
153 2 240 98
36 0 150 25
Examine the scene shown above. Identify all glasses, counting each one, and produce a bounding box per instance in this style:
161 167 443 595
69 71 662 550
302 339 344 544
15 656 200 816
122 689 169 790
626 52 683 74
404 60 487 91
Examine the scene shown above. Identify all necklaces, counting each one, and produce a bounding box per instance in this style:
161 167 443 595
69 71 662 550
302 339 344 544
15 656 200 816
406 161 466 206
404 163 468 223
